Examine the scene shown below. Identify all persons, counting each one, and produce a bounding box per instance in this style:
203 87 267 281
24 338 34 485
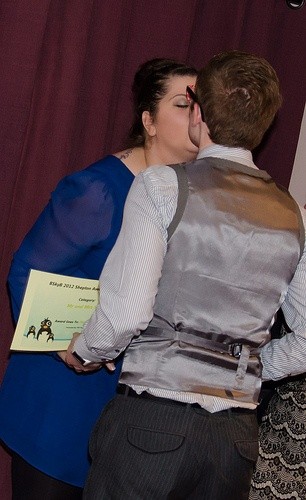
1 57 199 500
65 52 306 495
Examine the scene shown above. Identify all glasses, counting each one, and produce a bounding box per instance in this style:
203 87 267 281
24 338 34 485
185 84 205 122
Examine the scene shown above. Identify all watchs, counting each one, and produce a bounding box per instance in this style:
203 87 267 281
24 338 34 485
71 350 92 366
